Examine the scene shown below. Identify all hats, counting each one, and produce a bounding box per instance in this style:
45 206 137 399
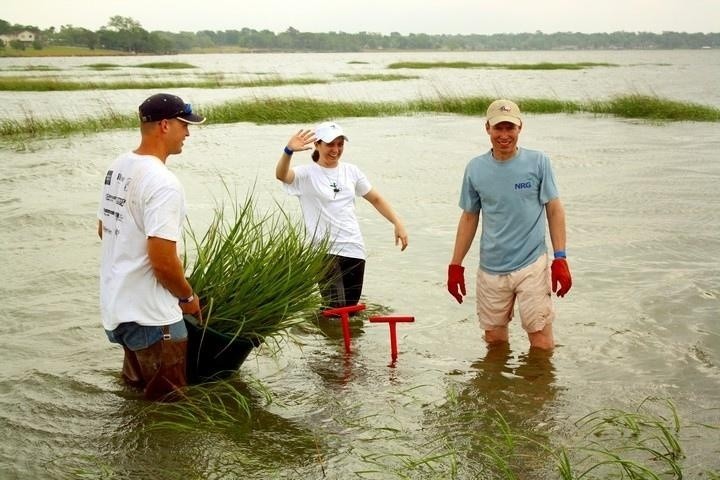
487 99 521 128
314 122 349 143
139 94 206 124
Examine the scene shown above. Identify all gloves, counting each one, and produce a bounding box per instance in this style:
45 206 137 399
551 258 571 297
447 265 466 304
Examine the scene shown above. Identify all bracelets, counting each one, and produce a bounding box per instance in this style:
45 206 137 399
554 251 567 258
180 292 194 304
284 146 294 155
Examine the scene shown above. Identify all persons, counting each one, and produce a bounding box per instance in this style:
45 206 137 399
447 98 572 351
97 94 207 394
276 122 410 320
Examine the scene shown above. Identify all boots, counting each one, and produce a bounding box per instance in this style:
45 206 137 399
122 338 188 402
